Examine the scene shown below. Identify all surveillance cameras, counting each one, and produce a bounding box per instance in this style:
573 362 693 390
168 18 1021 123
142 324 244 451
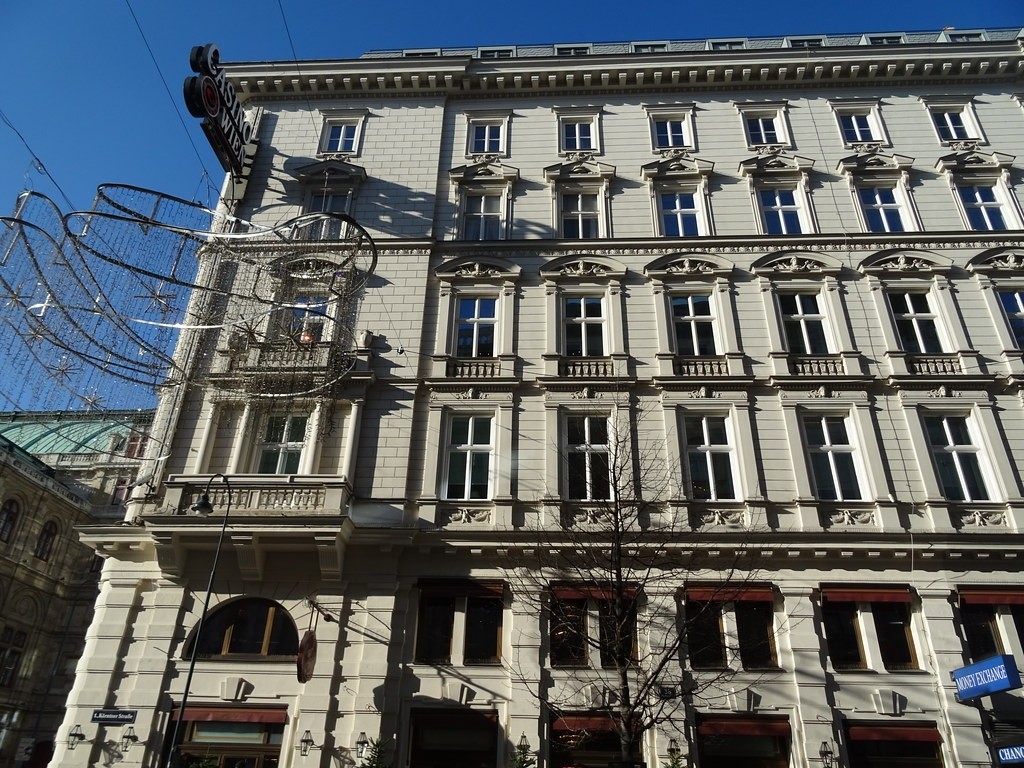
888 494 895 503
126 473 153 490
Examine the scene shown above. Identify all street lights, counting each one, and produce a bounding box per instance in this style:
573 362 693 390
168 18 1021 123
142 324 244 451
165 473 232 768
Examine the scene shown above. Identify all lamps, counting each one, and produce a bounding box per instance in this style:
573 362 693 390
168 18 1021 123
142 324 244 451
819 741 834 768
299 730 314 756
122 727 138 752
667 739 681 759
355 732 370 758
515 730 530 759
67 725 85 750
